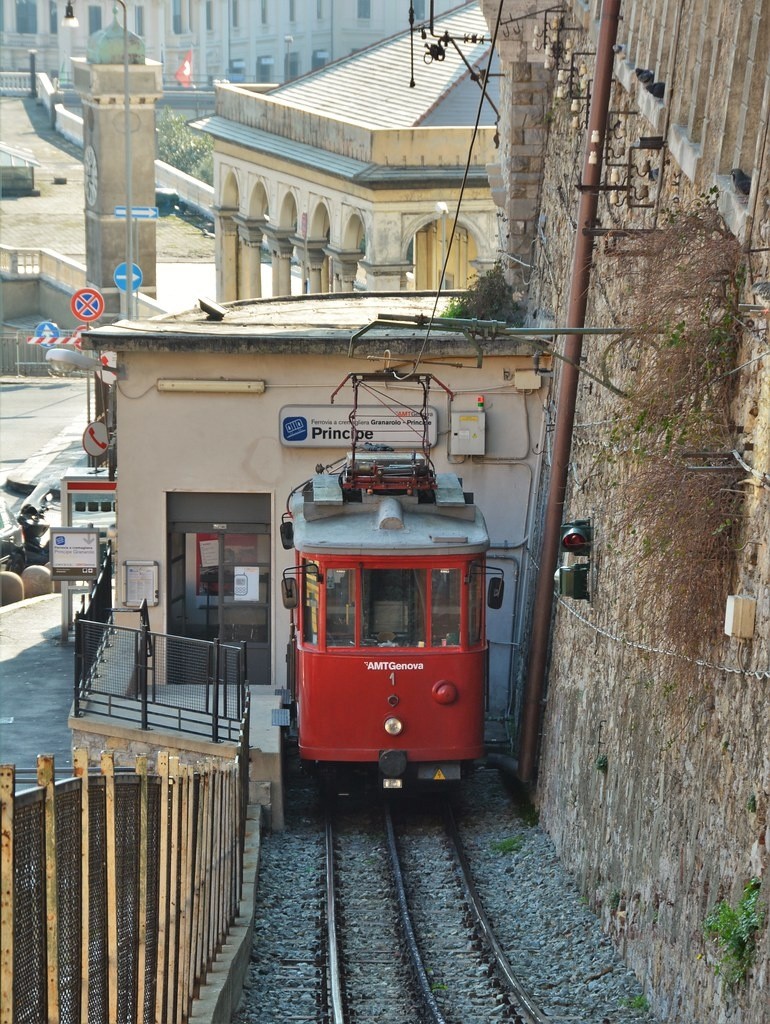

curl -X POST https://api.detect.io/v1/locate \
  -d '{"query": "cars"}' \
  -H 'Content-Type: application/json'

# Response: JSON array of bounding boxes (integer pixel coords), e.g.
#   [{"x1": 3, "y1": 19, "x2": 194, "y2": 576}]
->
[{"x1": 0, "y1": 495, "x2": 26, "y2": 561}]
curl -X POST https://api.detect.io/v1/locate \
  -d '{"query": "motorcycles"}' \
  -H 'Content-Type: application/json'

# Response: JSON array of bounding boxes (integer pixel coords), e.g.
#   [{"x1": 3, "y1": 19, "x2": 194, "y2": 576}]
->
[{"x1": 17, "y1": 493, "x2": 55, "y2": 562}]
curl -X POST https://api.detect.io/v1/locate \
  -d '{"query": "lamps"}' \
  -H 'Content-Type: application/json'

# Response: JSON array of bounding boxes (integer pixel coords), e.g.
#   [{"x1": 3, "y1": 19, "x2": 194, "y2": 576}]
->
[{"x1": 198, "y1": 299, "x2": 229, "y2": 320}]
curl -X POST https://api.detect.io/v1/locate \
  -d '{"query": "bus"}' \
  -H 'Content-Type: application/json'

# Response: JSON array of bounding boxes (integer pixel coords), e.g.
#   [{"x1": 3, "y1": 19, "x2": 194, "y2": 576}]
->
[{"x1": 268, "y1": 363, "x2": 492, "y2": 814}]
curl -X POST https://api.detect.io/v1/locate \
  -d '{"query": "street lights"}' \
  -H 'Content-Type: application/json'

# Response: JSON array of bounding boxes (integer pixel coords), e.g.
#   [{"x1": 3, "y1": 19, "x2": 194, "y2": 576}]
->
[{"x1": 284, "y1": 35, "x2": 293, "y2": 85}]
